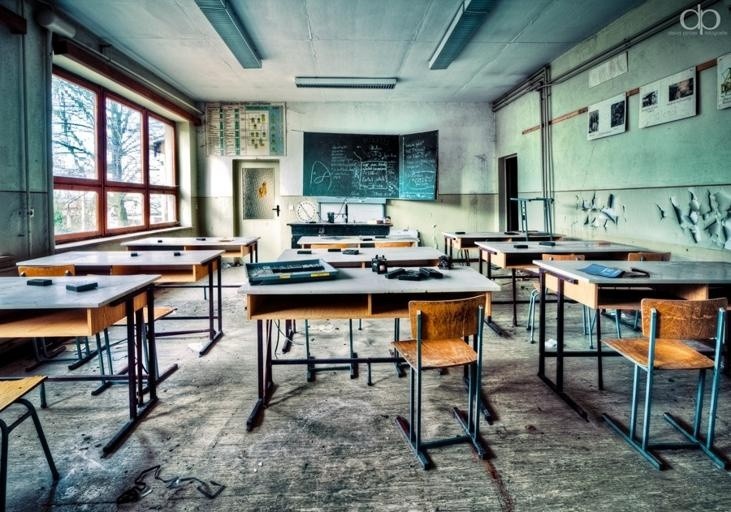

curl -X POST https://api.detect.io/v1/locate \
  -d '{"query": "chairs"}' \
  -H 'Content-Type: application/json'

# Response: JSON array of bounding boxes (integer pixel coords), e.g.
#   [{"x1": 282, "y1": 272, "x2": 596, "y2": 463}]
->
[
  {"x1": 0, "y1": 375, "x2": 62, "y2": 512},
  {"x1": 592, "y1": 252, "x2": 670, "y2": 339},
  {"x1": 18, "y1": 265, "x2": 106, "y2": 370},
  {"x1": 308, "y1": 243, "x2": 363, "y2": 331},
  {"x1": 510, "y1": 197, "x2": 554, "y2": 273},
  {"x1": 597, "y1": 297, "x2": 728, "y2": 473},
  {"x1": 374, "y1": 242, "x2": 416, "y2": 248},
  {"x1": 90, "y1": 306, "x2": 178, "y2": 397},
  {"x1": 527, "y1": 253, "x2": 594, "y2": 350},
  {"x1": 388, "y1": 293, "x2": 487, "y2": 470}
]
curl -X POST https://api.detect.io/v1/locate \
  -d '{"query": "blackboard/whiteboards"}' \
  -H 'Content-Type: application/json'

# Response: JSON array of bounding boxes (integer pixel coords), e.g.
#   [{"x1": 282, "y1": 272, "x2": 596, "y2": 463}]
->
[{"x1": 302, "y1": 130, "x2": 439, "y2": 202}]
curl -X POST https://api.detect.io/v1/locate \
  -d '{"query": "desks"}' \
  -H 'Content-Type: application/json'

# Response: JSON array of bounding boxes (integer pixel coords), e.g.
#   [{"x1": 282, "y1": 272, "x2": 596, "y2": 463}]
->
[
  {"x1": 120, "y1": 236, "x2": 260, "y2": 311},
  {"x1": 237, "y1": 235, "x2": 501, "y2": 439},
  {"x1": 16, "y1": 251, "x2": 227, "y2": 372},
  {"x1": 444, "y1": 231, "x2": 731, "y2": 426},
  {"x1": 1, "y1": 273, "x2": 162, "y2": 454}
]
